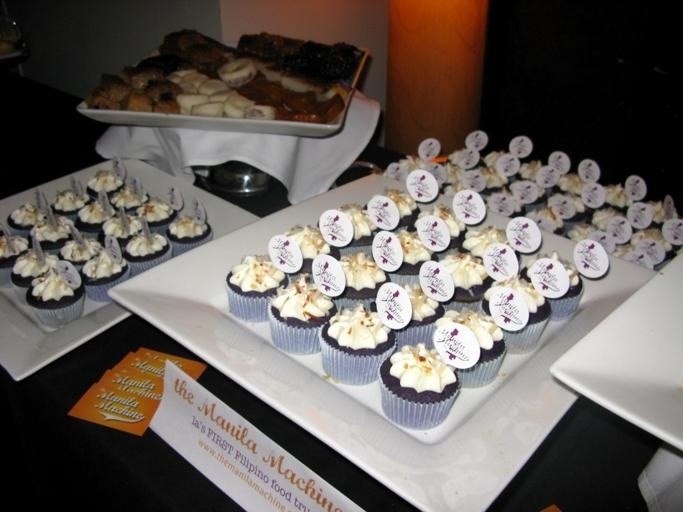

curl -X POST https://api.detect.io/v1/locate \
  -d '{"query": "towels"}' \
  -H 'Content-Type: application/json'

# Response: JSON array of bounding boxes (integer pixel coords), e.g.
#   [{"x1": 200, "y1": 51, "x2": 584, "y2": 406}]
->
[{"x1": 93, "y1": 84, "x2": 380, "y2": 204}]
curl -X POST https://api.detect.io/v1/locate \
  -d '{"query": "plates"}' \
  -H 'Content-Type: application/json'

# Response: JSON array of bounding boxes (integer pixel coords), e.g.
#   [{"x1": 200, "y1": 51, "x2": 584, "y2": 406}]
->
[
  {"x1": 76, "y1": 45, "x2": 372, "y2": 140},
  {"x1": 0, "y1": 156, "x2": 262, "y2": 386},
  {"x1": 105, "y1": 172, "x2": 660, "y2": 512},
  {"x1": 549, "y1": 253, "x2": 683, "y2": 449}
]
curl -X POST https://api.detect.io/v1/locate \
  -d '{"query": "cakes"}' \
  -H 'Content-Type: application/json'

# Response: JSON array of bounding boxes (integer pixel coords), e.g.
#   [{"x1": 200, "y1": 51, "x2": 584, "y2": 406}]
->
[
  {"x1": 0, "y1": 168, "x2": 212, "y2": 322},
  {"x1": 89, "y1": 27, "x2": 366, "y2": 125}
]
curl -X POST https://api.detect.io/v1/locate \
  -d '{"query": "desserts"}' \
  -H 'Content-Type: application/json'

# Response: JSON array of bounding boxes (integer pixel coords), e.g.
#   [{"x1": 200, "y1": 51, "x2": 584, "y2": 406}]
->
[
  {"x1": 382, "y1": 149, "x2": 680, "y2": 272},
  {"x1": 225, "y1": 187, "x2": 583, "y2": 429}
]
